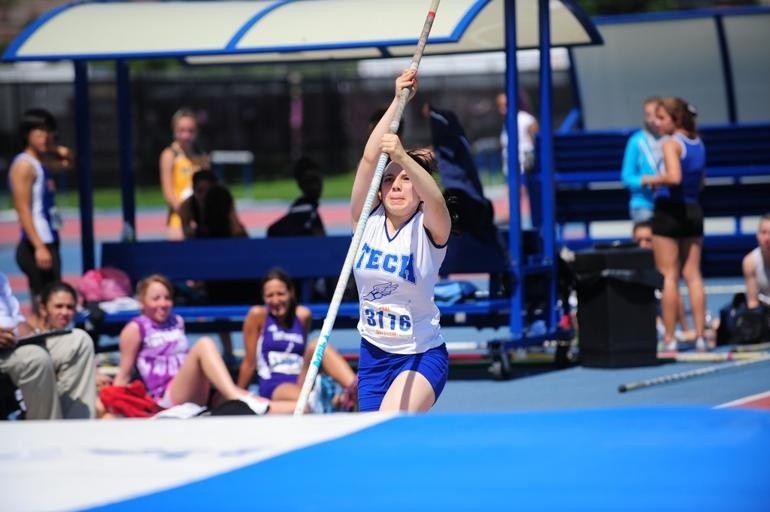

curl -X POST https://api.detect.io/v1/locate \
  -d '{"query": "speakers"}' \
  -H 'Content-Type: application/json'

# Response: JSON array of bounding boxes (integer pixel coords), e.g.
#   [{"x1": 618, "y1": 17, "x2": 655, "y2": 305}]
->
[{"x1": 573, "y1": 247, "x2": 658, "y2": 369}]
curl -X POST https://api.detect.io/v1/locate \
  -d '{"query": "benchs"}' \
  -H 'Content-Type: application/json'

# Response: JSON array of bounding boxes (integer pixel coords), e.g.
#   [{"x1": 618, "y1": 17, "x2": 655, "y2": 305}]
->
[
  {"x1": 527, "y1": 120, "x2": 769, "y2": 223},
  {"x1": 93, "y1": 228, "x2": 559, "y2": 373}
]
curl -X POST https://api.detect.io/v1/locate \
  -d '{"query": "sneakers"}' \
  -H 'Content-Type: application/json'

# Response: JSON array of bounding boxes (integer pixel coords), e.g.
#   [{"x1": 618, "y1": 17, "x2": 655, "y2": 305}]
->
[
  {"x1": 694, "y1": 335, "x2": 707, "y2": 352},
  {"x1": 307, "y1": 374, "x2": 331, "y2": 413},
  {"x1": 342, "y1": 373, "x2": 358, "y2": 410},
  {"x1": 238, "y1": 391, "x2": 270, "y2": 415},
  {"x1": 657, "y1": 338, "x2": 678, "y2": 352}
]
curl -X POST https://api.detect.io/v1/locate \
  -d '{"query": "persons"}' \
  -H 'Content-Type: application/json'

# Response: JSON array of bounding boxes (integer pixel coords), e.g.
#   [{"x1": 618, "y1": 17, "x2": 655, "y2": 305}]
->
[
  {"x1": 155, "y1": 101, "x2": 218, "y2": 238},
  {"x1": 174, "y1": 169, "x2": 245, "y2": 245},
  {"x1": 8, "y1": 110, "x2": 75, "y2": 292},
  {"x1": 228, "y1": 271, "x2": 358, "y2": 413},
  {"x1": 112, "y1": 272, "x2": 272, "y2": 414},
  {"x1": 490, "y1": 92, "x2": 536, "y2": 218},
  {"x1": 344, "y1": 61, "x2": 454, "y2": 413},
  {"x1": 618, "y1": 96, "x2": 718, "y2": 355},
  {"x1": 741, "y1": 216, "x2": 770, "y2": 333},
  {"x1": 1, "y1": 277, "x2": 106, "y2": 419}
]
